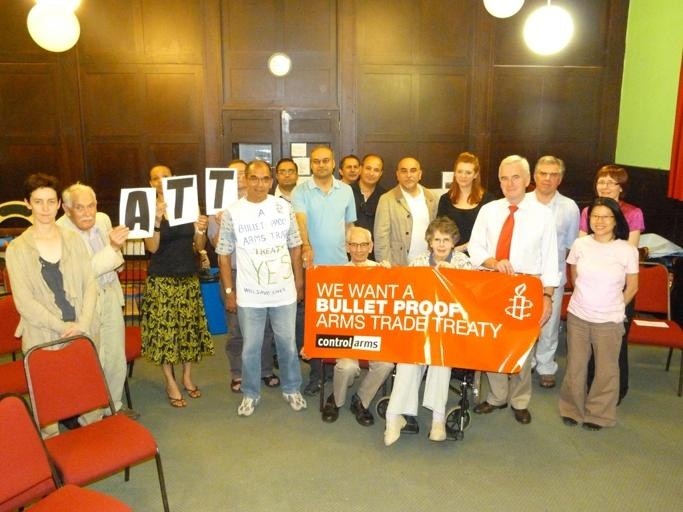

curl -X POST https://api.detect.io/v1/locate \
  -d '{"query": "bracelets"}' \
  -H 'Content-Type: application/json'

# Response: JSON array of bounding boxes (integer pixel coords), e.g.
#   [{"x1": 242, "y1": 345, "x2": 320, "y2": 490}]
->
[
  {"x1": 197, "y1": 228, "x2": 207, "y2": 235},
  {"x1": 154, "y1": 226, "x2": 161, "y2": 232}
]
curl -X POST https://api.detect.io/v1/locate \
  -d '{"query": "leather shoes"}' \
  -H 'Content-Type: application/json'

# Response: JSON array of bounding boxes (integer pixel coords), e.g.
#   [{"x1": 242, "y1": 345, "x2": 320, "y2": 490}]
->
[
  {"x1": 322, "y1": 393, "x2": 338, "y2": 422},
  {"x1": 473, "y1": 401, "x2": 508, "y2": 414},
  {"x1": 350, "y1": 394, "x2": 374, "y2": 426},
  {"x1": 511, "y1": 406, "x2": 531, "y2": 424}
]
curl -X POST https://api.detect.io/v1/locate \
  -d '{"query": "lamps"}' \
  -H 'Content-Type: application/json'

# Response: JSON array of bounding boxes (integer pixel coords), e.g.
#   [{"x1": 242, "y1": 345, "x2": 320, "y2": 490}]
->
[{"x1": 522, "y1": 0, "x2": 575, "y2": 56}]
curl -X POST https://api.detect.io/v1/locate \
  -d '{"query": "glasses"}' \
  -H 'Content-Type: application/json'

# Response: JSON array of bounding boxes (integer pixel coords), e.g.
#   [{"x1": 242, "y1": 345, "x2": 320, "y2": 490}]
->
[
  {"x1": 349, "y1": 242, "x2": 369, "y2": 248},
  {"x1": 592, "y1": 215, "x2": 615, "y2": 221},
  {"x1": 597, "y1": 180, "x2": 620, "y2": 187}
]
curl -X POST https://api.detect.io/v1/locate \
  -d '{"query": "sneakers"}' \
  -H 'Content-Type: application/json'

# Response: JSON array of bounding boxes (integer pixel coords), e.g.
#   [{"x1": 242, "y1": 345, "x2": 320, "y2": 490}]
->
[
  {"x1": 563, "y1": 417, "x2": 602, "y2": 431},
  {"x1": 119, "y1": 406, "x2": 140, "y2": 418},
  {"x1": 540, "y1": 374, "x2": 555, "y2": 388},
  {"x1": 383, "y1": 415, "x2": 407, "y2": 446},
  {"x1": 304, "y1": 376, "x2": 329, "y2": 396},
  {"x1": 282, "y1": 391, "x2": 307, "y2": 411},
  {"x1": 429, "y1": 421, "x2": 446, "y2": 442},
  {"x1": 238, "y1": 394, "x2": 261, "y2": 416}
]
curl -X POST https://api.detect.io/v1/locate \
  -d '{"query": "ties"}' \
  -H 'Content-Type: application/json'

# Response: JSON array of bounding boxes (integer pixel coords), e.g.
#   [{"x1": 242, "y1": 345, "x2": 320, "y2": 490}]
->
[{"x1": 495, "y1": 206, "x2": 519, "y2": 260}]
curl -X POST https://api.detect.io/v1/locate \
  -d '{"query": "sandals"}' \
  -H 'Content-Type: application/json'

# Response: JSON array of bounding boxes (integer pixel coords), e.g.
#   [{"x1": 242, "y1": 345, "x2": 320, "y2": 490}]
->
[
  {"x1": 263, "y1": 374, "x2": 280, "y2": 387},
  {"x1": 231, "y1": 379, "x2": 242, "y2": 393},
  {"x1": 166, "y1": 387, "x2": 187, "y2": 407},
  {"x1": 185, "y1": 385, "x2": 200, "y2": 398}
]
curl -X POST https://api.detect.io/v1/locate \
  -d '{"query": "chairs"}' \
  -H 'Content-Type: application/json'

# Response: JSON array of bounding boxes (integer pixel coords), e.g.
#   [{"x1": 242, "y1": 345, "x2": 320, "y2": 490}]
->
[
  {"x1": 24, "y1": 335, "x2": 170, "y2": 512},
  {"x1": 1, "y1": 293, "x2": 28, "y2": 396},
  {"x1": 0, "y1": 393, "x2": 133, "y2": 512},
  {"x1": 125, "y1": 326, "x2": 142, "y2": 408},
  {"x1": 561, "y1": 264, "x2": 573, "y2": 328},
  {"x1": 627, "y1": 262, "x2": 683, "y2": 396}
]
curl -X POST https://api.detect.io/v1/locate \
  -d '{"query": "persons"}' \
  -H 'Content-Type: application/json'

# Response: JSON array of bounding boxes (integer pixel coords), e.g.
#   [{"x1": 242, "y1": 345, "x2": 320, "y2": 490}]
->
[
  {"x1": 469, "y1": 155, "x2": 564, "y2": 425},
  {"x1": 143, "y1": 159, "x2": 208, "y2": 408},
  {"x1": 290, "y1": 144, "x2": 357, "y2": 396},
  {"x1": 508, "y1": 156, "x2": 580, "y2": 387},
  {"x1": 339, "y1": 155, "x2": 362, "y2": 183},
  {"x1": 436, "y1": 152, "x2": 498, "y2": 257},
  {"x1": 558, "y1": 196, "x2": 639, "y2": 431},
  {"x1": 193, "y1": 241, "x2": 210, "y2": 268},
  {"x1": 347, "y1": 153, "x2": 387, "y2": 262},
  {"x1": 6, "y1": 173, "x2": 102, "y2": 440},
  {"x1": 580, "y1": 165, "x2": 644, "y2": 406},
  {"x1": 383, "y1": 215, "x2": 475, "y2": 445},
  {"x1": 206, "y1": 159, "x2": 280, "y2": 393},
  {"x1": 322, "y1": 227, "x2": 395, "y2": 425},
  {"x1": 215, "y1": 159, "x2": 308, "y2": 416},
  {"x1": 54, "y1": 181, "x2": 142, "y2": 420},
  {"x1": 374, "y1": 155, "x2": 438, "y2": 266}
]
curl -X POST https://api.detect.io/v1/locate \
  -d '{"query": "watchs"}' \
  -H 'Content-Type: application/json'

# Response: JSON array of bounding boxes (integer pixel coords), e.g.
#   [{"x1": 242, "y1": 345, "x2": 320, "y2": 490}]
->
[
  {"x1": 543, "y1": 292, "x2": 554, "y2": 302},
  {"x1": 463, "y1": 245, "x2": 467, "y2": 251},
  {"x1": 225, "y1": 287, "x2": 235, "y2": 294}
]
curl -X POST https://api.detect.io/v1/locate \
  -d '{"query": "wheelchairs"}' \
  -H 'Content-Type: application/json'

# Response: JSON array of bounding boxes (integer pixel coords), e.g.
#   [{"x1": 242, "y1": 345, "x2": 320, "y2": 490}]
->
[{"x1": 374, "y1": 364, "x2": 484, "y2": 443}]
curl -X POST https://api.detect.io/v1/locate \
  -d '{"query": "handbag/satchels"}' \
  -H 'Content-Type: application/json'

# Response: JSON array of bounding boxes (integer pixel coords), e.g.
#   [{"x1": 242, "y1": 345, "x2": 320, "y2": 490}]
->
[{"x1": 194, "y1": 249, "x2": 210, "y2": 272}]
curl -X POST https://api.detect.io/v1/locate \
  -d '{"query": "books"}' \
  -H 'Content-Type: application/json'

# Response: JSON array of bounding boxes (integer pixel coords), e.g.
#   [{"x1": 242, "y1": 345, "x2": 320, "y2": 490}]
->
[{"x1": 117, "y1": 238, "x2": 150, "y2": 326}]
